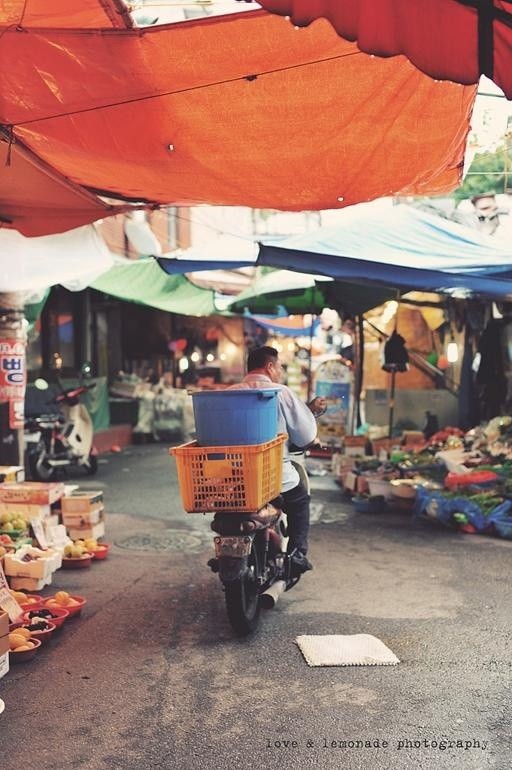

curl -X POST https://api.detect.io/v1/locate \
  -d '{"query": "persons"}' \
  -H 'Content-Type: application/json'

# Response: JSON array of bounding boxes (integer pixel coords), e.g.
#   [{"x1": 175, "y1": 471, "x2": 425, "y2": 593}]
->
[
  {"x1": 310, "y1": 397, "x2": 327, "y2": 412},
  {"x1": 224, "y1": 346, "x2": 317, "y2": 570}
]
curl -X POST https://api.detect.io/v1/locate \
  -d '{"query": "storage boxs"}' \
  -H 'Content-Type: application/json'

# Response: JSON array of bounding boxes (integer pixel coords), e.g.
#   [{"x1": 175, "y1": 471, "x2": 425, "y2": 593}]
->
[{"x1": 0, "y1": 480, "x2": 105, "y2": 541}]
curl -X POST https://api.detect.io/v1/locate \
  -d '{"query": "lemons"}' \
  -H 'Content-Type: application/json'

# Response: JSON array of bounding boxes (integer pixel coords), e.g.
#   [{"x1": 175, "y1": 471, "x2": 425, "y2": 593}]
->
[
  {"x1": 10, "y1": 589, "x2": 36, "y2": 605},
  {"x1": 64, "y1": 537, "x2": 104, "y2": 558}
]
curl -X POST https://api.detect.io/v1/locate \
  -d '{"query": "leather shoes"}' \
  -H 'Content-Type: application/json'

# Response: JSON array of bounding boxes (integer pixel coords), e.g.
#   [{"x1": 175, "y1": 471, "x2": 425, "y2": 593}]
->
[{"x1": 291, "y1": 551, "x2": 313, "y2": 570}]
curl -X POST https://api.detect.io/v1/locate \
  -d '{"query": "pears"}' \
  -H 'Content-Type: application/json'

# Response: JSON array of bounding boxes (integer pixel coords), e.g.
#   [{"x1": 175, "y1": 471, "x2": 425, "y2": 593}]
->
[{"x1": 0, "y1": 510, "x2": 27, "y2": 531}]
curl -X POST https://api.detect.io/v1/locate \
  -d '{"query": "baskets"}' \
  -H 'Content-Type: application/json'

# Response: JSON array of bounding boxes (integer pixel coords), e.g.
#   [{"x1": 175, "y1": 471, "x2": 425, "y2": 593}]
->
[{"x1": 168, "y1": 432, "x2": 288, "y2": 513}]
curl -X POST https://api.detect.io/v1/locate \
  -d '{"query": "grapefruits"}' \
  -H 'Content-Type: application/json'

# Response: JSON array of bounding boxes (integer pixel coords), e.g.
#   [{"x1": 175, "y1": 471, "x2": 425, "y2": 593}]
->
[{"x1": 9, "y1": 628, "x2": 35, "y2": 651}]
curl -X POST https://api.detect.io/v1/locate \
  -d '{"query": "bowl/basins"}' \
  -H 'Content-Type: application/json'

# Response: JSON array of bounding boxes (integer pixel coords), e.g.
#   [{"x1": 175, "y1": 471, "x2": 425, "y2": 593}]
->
[
  {"x1": 61, "y1": 552, "x2": 95, "y2": 569},
  {"x1": 352, "y1": 496, "x2": 394, "y2": 514},
  {"x1": 491, "y1": 515, "x2": 511, "y2": 537},
  {"x1": 389, "y1": 478, "x2": 425, "y2": 499},
  {"x1": 19, "y1": 593, "x2": 43, "y2": 610},
  {"x1": 367, "y1": 479, "x2": 395, "y2": 501},
  {"x1": 9, "y1": 620, "x2": 56, "y2": 643},
  {"x1": 7, "y1": 633, "x2": 42, "y2": 666},
  {"x1": 39, "y1": 594, "x2": 87, "y2": 619},
  {"x1": 19, "y1": 607, "x2": 70, "y2": 631},
  {"x1": 83, "y1": 543, "x2": 110, "y2": 561}
]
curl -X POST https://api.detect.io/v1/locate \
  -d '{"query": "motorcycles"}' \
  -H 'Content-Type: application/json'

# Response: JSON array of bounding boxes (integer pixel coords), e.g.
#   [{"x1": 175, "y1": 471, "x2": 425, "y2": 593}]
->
[
  {"x1": 207, "y1": 395, "x2": 329, "y2": 634},
  {"x1": 24, "y1": 360, "x2": 98, "y2": 482}
]
